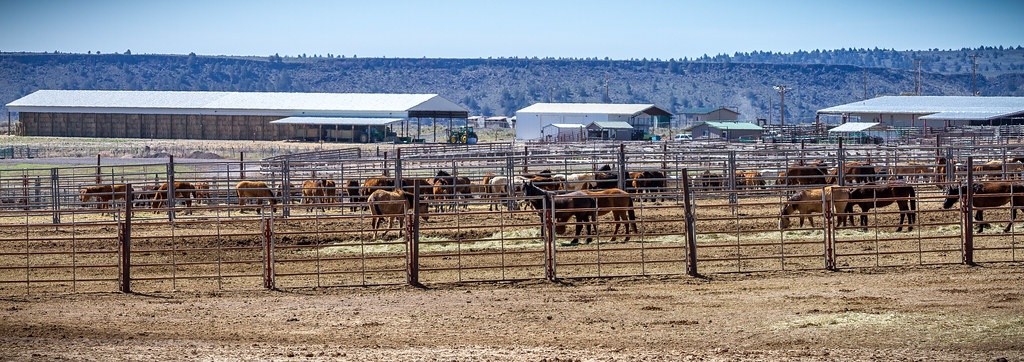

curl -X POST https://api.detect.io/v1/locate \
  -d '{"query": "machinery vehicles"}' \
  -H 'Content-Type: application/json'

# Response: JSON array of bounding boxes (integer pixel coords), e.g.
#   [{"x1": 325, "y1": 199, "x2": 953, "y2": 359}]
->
[
  {"x1": 446, "y1": 126, "x2": 478, "y2": 144},
  {"x1": 359, "y1": 127, "x2": 384, "y2": 143}
]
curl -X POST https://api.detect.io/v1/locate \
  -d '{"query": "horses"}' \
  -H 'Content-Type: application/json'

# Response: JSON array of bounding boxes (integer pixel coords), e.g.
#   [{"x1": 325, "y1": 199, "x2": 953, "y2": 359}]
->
[{"x1": 79, "y1": 160, "x2": 1023, "y2": 245}]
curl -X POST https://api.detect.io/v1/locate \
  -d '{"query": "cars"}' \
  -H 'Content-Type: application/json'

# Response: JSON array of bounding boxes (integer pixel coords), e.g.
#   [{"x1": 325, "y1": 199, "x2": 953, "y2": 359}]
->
[{"x1": 674, "y1": 134, "x2": 692, "y2": 140}]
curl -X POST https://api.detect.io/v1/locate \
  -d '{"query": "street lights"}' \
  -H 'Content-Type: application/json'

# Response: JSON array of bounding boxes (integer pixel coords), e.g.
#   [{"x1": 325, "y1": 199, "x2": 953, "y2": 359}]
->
[{"x1": 773, "y1": 84, "x2": 792, "y2": 143}]
